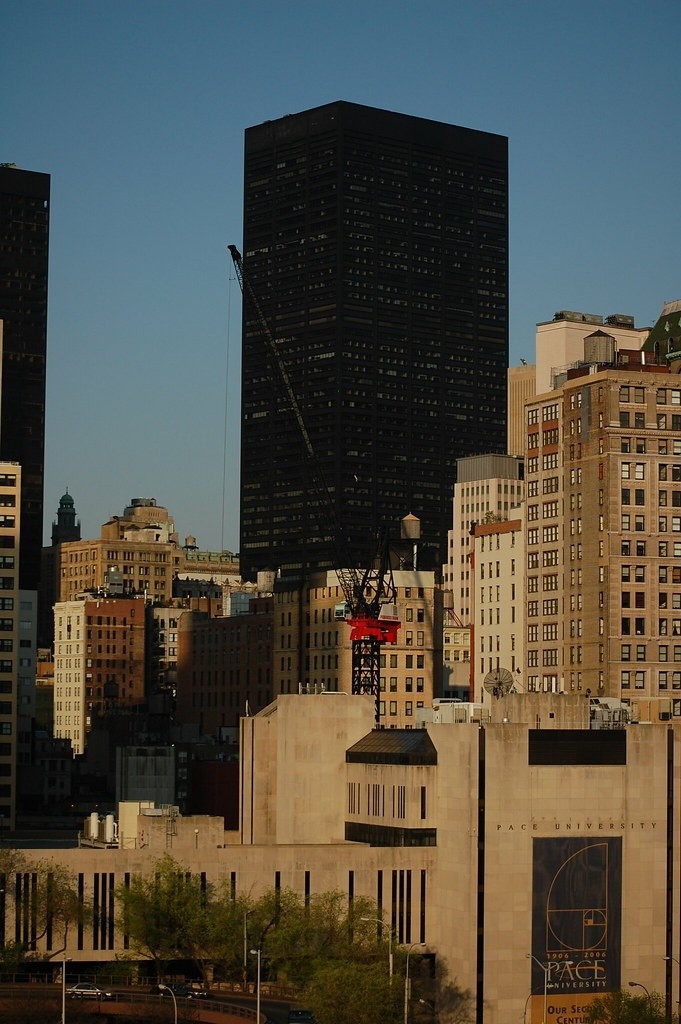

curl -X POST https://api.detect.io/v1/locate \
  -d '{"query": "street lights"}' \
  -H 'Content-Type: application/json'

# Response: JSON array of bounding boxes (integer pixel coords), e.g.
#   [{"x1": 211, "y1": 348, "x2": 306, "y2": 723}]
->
[
  {"x1": 230, "y1": 898, "x2": 247, "y2": 991},
  {"x1": 62, "y1": 952, "x2": 72, "y2": 1024},
  {"x1": 158, "y1": 984, "x2": 178, "y2": 1024},
  {"x1": 361, "y1": 917, "x2": 394, "y2": 993},
  {"x1": 403, "y1": 943, "x2": 427, "y2": 1022},
  {"x1": 250, "y1": 949, "x2": 260, "y2": 1024}
]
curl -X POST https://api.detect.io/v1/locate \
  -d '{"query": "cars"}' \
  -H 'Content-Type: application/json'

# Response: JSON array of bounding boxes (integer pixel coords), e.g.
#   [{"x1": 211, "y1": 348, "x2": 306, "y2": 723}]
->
[
  {"x1": 65, "y1": 982, "x2": 113, "y2": 1000},
  {"x1": 288, "y1": 1007, "x2": 316, "y2": 1024},
  {"x1": 149, "y1": 983, "x2": 209, "y2": 1000},
  {"x1": 62, "y1": 795, "x2": 99, "y2": 815}
]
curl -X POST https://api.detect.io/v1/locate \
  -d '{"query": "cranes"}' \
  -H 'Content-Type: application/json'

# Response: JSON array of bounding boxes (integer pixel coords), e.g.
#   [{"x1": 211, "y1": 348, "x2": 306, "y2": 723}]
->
[{"x1": 226, "y1": 241, "x2": 411, "y2": 728}]
